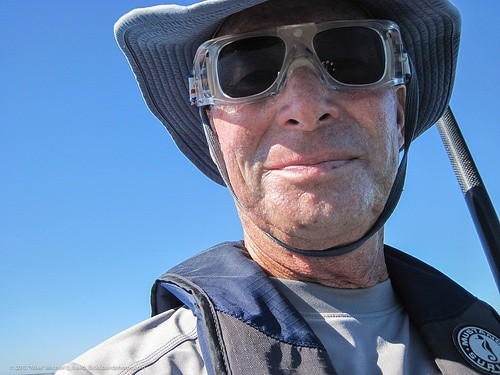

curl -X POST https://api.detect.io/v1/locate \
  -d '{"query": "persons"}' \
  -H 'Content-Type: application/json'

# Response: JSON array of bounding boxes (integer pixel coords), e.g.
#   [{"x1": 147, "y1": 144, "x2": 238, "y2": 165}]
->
[{"x1": 47, "y1": 1, "x2": 500, "y2": 374}]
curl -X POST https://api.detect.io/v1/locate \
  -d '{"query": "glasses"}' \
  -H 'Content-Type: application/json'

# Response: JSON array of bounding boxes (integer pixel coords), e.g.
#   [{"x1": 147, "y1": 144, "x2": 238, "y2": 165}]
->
[{"x1": 186, "y1": 17, "x2": 409, "y2": 105}]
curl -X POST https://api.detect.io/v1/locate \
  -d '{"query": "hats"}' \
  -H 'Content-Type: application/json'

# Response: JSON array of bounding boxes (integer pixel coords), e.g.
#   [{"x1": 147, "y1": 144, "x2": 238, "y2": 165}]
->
[{"x1": 114, "y1": 0, "x2": 463, "y2": 186}]
